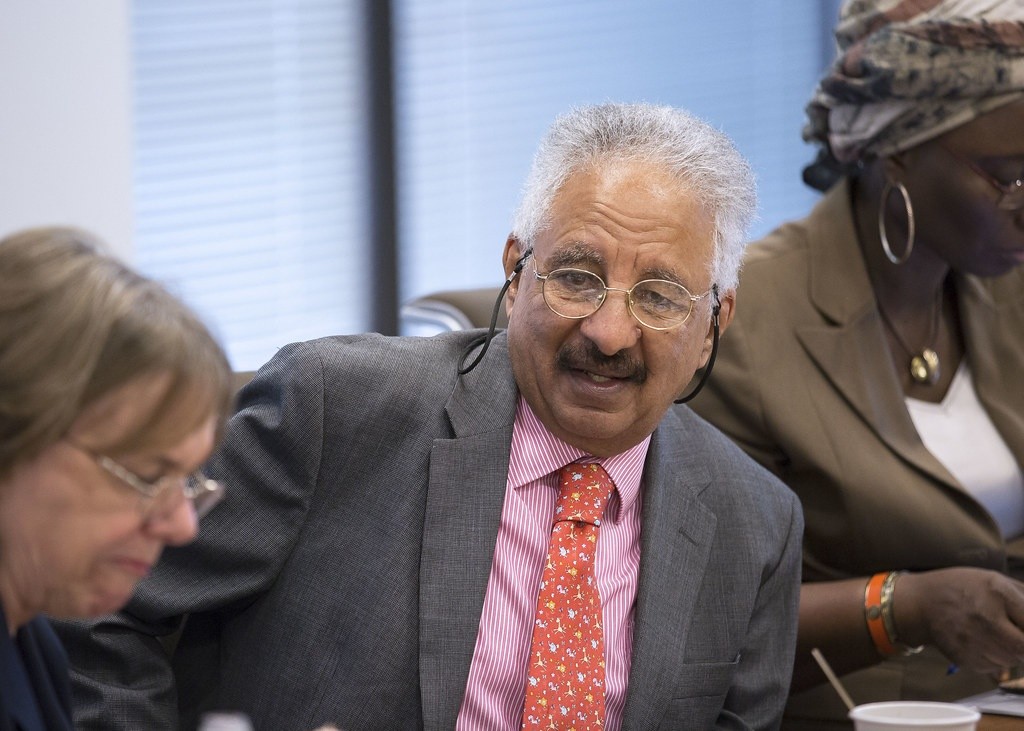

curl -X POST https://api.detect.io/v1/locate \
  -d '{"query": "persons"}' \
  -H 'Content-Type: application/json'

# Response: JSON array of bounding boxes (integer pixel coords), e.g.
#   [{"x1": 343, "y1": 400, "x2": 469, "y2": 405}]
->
[
  {"x1": 673, "y1": 0, "x2": 1024, "y2": 731},
  {"x1": 52, "y1": 102, "x2": 803, "y2": 731},
  {"x1": 0, "y1": 226, "x2": 235, "y2": 731}
]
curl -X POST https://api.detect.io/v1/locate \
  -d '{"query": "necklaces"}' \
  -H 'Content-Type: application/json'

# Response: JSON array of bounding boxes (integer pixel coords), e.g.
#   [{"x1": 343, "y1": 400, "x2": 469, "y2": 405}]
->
[{"x1": 869, "y1": 286, "x2": 940, "y2": 386}]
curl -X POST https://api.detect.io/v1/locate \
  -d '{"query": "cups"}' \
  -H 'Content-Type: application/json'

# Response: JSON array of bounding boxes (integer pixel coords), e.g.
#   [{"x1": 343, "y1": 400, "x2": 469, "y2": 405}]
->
[{"x1": 848, "y1": 700, "x2": 982, "y2": 731}]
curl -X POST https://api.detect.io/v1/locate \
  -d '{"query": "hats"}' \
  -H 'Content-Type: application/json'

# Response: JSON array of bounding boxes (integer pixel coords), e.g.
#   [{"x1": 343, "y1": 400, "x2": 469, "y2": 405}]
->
[{"x1": 798, "y1": 0, "x2": 1023, "y2": 192}]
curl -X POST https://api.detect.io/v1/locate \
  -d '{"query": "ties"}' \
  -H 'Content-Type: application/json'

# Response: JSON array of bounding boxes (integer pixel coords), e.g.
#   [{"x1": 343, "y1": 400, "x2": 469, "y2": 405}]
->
[{"x1": 523, "y1": 461, "x2": 615, "y2": 731}]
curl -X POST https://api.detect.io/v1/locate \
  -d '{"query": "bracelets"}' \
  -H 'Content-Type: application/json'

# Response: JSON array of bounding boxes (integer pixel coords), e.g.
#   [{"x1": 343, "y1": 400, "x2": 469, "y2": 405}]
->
[{"x1": 864, "y1": 571, "x2": 924, "y2": 662}]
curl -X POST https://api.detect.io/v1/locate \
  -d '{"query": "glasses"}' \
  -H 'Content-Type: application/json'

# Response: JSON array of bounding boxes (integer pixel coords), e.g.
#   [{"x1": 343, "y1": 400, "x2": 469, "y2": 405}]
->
[
  {"x1": 528, "y1": 245, "x2": 722, "y2": 330},
  {"x1": 58, "y1": 428, "x2": 224, "y2": 519},
  {"x1": 930, "y1": 135, "x2": 1023, "y2": 212}
]
